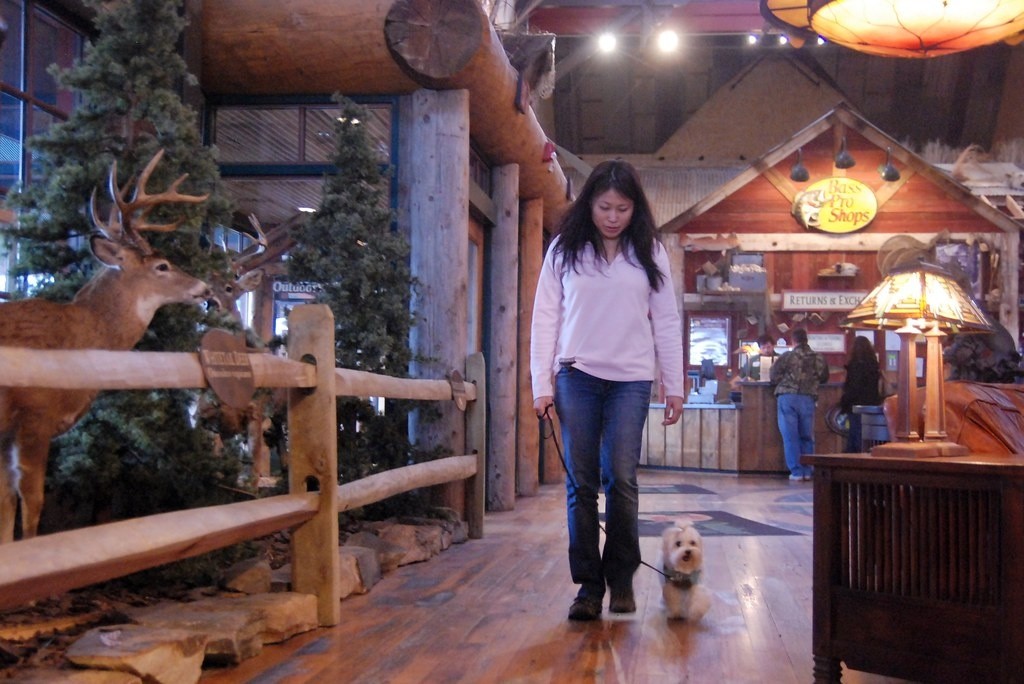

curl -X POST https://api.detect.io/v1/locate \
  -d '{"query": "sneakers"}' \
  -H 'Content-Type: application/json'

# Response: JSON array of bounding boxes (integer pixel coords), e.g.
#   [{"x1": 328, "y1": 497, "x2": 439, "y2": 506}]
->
[
  {"x1": 569, "y1": 581, "x2": 606, "y2": 617},
  {"x1": 610, "y1": 583, "x2": 635, "y2": 613}
]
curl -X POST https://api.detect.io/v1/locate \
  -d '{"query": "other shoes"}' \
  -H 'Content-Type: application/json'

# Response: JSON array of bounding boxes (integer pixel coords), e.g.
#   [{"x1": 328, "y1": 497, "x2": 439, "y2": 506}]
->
[
  {"x1": 789, "y1": 474, "x2": 803, "y2": 481},
  {"x1": 803, "y1": 476, "x2": 810, "y2": 480}
]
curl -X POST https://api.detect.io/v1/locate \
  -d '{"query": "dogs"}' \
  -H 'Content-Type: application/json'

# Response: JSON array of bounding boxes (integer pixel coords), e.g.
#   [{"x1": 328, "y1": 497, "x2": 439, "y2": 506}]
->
[{"x1": 656, "y1": 528, "x2": 713, "y2": 626}]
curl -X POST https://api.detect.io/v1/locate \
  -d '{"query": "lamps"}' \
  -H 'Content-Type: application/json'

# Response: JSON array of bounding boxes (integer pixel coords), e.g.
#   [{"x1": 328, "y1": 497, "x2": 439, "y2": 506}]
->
[
  {"x1": 836, "y1": 256, "x2": 995, "y2": 454},
  {"x1": 791, "y1": 148, "x2": 810, "y2": 182},
  {"x1": 879, "y1": 146, "x2": 901, "y2": 181},
  {"x1": 835, "y1": 137, "x2": 856, "y2": 169}
]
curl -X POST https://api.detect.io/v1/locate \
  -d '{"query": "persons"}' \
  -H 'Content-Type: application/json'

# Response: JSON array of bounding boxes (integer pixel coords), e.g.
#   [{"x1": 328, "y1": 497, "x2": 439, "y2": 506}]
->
[
  {"x1": 531, "y1": 160, "x2": 687, "y2": 621},
  {"x1": 769, "y1": 329, "x2": 830, "y2": 482},
  {"x1": 943, "y1": 313, "x2": 1024, "y2": 455},
  {"x1": 839, "y1": 335, "x2": 887, "y2": 453},
  {"x1": 729, "y1": 334, "x2": 781, "y2": 390}
]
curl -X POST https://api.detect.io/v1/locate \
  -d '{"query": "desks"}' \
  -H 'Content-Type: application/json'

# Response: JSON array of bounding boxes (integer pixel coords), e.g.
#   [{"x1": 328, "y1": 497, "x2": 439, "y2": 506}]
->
[{"x1": 798, "y1": 452, "x2": 1024, "y2": 684}]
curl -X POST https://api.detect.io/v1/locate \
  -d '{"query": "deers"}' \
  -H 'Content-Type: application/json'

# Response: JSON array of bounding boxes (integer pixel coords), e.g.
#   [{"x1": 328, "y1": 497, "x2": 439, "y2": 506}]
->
[
  {"x1": 186, "y1": 211, "x2": 263, "y2": 498},
  {"x1": 0, "y1": 150, "x2": 216, "y2": 611}
]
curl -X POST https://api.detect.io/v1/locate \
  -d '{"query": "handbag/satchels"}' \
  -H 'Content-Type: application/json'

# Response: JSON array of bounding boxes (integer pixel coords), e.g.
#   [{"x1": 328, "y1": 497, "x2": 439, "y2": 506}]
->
[{"x1": 878, "y1": 370, "x2": 895, "y2": 399}]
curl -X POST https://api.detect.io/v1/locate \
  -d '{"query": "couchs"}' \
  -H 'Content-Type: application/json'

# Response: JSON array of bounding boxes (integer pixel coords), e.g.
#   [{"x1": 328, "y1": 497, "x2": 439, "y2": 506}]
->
[{"x1": 883, "y1": 378, "x2": 1024, "y2": 456}]
